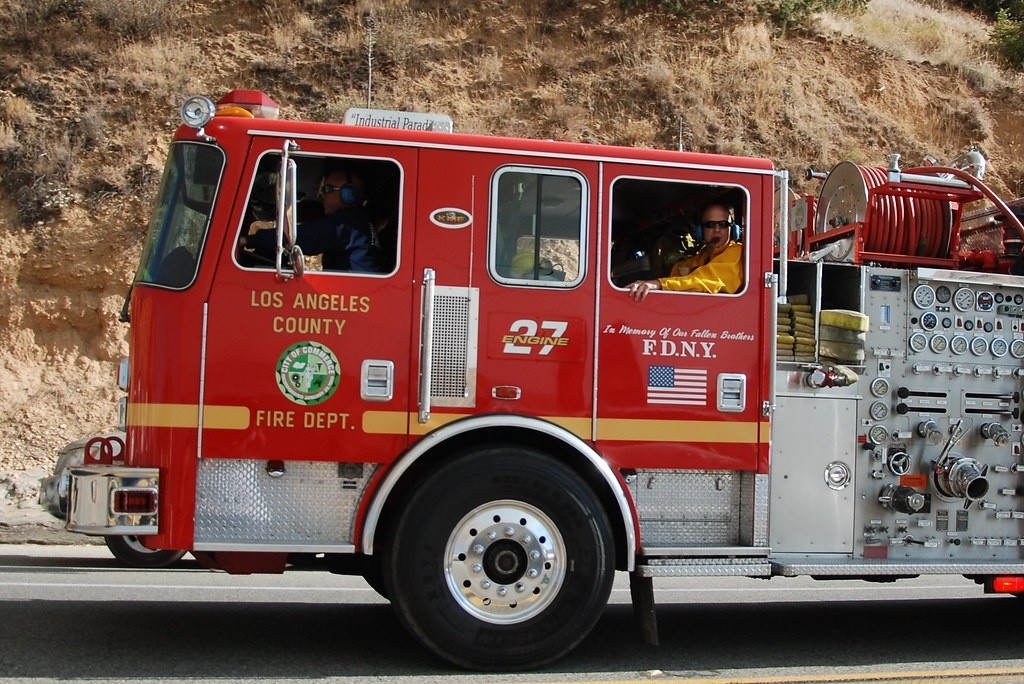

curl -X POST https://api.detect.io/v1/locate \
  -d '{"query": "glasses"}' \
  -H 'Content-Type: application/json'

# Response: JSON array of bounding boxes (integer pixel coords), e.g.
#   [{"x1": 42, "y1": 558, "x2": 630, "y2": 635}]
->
[
  {"x1": 321, "y1": 184, "x2": 342, "y2": 194},
  {"x1": 702, "y1": 220, "x2": 733, "y2": 228}
]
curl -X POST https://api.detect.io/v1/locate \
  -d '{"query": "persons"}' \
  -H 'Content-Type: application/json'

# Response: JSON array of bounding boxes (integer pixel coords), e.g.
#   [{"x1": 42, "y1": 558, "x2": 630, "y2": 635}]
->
[
  {"x1": 182, "y1": 162, "x2": 384, "y2": 274},
  {"x1": 624, "y1": 204, "x2": 743, "y2": 302}
]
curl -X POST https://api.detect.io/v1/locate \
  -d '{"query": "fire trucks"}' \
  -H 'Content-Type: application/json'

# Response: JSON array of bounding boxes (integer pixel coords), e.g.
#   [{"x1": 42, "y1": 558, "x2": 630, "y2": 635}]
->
[{"x1": 35, "y1": 90, "x2": 1024, "y2": 674}]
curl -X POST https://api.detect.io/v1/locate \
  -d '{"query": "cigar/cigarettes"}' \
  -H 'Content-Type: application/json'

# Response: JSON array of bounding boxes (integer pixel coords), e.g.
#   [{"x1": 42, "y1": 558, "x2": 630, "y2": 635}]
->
[{"x1": 707, "y1": 240, "x2": 718, "y2": 246}]
